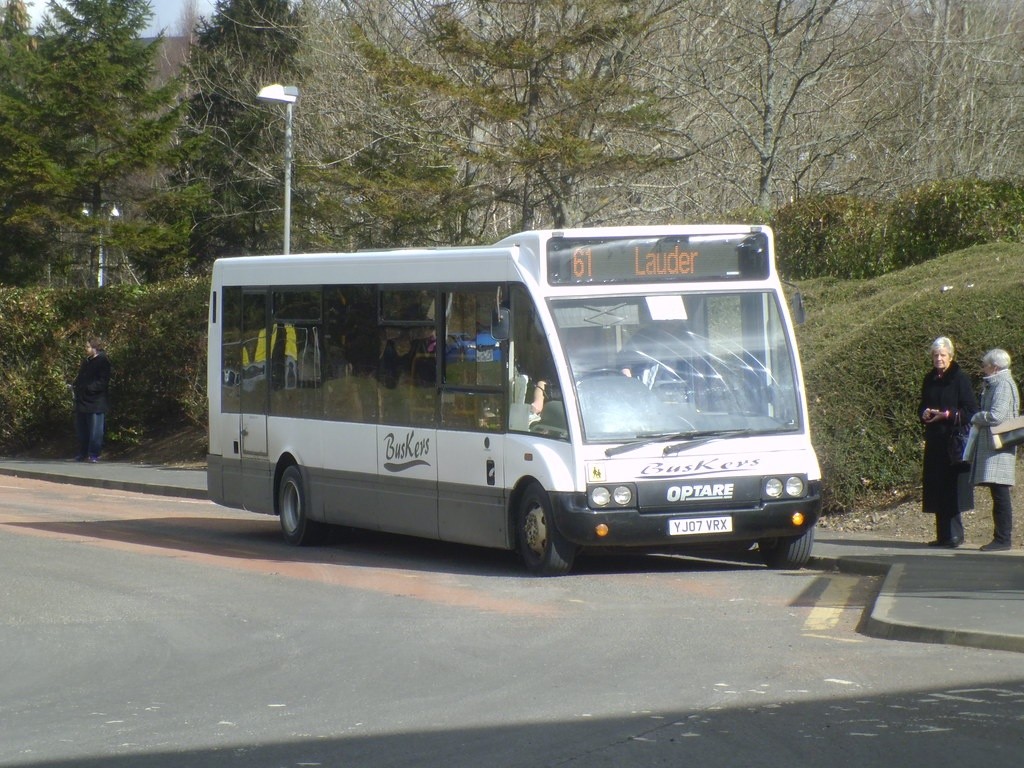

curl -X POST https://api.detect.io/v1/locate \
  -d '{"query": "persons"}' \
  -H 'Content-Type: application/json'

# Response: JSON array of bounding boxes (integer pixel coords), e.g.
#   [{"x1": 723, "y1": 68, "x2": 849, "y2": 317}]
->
[
  {"x1": 615, "y1": 319, "x2": 688, "y2": 405},
  {"x1": 529, "y1": 336, "x2": 592, "y2": 415},
  {"x1": 916, "y1": 337, "x2": 978, "y2": 547},
  {"x1": 64, "y1": 337, "x2": 111, "y2": 463},
  {"x1": 972, "y1": 349, "x2": 1020, "y2": 552}
]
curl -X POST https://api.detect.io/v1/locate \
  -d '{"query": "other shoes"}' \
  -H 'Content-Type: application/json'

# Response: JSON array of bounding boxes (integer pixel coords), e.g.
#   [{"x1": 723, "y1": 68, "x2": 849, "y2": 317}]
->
[
  {"x1": 980, "y1": 541, "x2": 1010, "y2": 551},
  {"x1": 948, "y1": 536, "x2": 964, "y2": 548},
  {"x1": 76, "y1": 456, "x2": 86, "y2": 463},
  {"x1": 928, "y1": 540, "x2": 949, "y2": 546},
  {"x1": 90, "y1": 457, "x2": 97, "y2": 463}
]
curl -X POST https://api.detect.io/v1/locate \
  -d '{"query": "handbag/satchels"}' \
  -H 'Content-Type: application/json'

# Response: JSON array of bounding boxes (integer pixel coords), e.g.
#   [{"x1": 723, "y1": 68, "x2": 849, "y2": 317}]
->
[
  {"x1": 949, "y1": 412, "x2": 971, "y2": 466},
  {"x1": 989, "y1": 380, "x2": 1024, "y2": 450},
  {"x1": 962, "y1": 423, "x2": 977, "y2": 464}
]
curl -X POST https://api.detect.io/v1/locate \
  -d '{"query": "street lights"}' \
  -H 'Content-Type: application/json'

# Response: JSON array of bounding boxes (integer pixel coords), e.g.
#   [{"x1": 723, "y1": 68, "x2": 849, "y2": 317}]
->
[
  {"x1": 80, "y1": 201, "x2": 121, "y2": 288},
  {"x1": 254, "y1": 81, "x2": 298, "y2": 254}
]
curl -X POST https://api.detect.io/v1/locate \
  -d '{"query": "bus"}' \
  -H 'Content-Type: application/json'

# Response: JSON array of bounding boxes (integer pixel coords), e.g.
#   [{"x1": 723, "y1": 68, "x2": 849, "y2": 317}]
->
[{"x1": 205, "y1": 222, "x2": 826, "y2": 576}]
